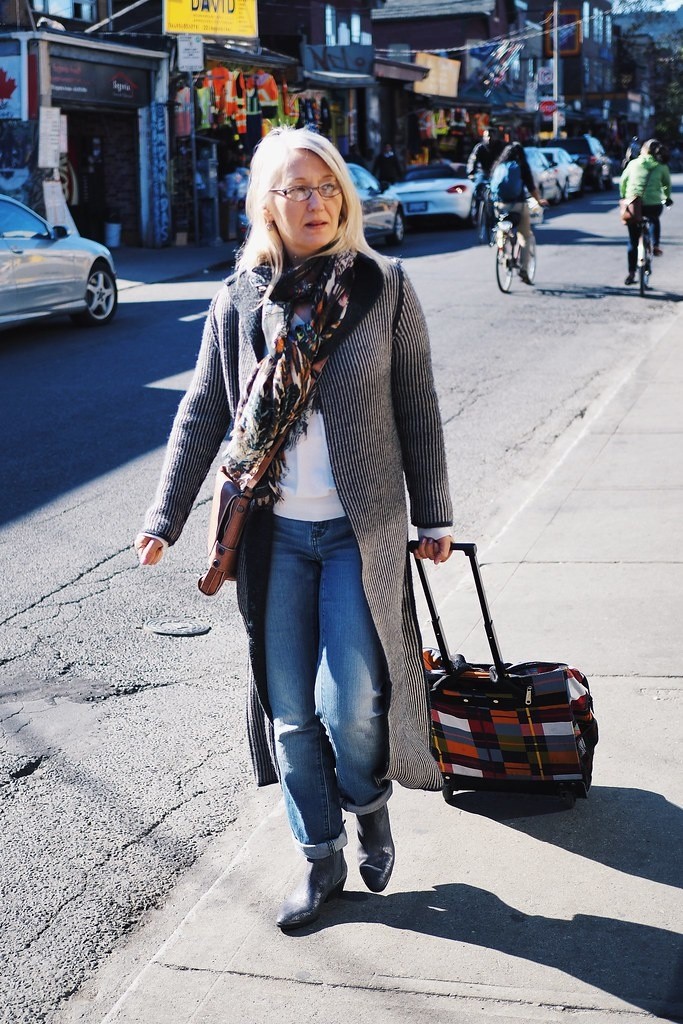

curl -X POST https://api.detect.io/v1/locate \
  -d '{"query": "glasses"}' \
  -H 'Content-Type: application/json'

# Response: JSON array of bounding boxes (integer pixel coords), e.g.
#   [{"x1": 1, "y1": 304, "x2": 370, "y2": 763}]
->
[{"x1": 269, "y1": 183, "x2": 342, "y2": 202}]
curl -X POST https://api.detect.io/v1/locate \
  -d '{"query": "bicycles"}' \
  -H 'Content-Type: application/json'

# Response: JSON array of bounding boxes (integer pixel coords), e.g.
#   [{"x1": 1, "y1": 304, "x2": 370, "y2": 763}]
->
[
  {"x1": 638, "y1": 200, "x2": 671, "y2": 297},
  {"x1": 493, "y1": 202, "x2": 548, "y2": 293}
]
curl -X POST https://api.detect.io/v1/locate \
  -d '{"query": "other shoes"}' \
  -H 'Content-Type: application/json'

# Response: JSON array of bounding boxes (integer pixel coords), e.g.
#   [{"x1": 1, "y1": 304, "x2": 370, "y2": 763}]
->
[
  {"x1": 518, "y1": 270, "x2": 531, "y2": 285},
  {"x1": 625, "y1": 273, "x2": 634, "y2": 285},
  {"x1": 653, "y1": 249, "x2": 663, "y2": 257}
]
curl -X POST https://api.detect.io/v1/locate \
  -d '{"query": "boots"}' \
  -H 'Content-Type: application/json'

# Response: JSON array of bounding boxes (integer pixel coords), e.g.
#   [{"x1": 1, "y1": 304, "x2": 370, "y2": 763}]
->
[
  {"x1": 276, "y1": 848, "x2": 347, "y2": 930},
  {"x1": 356, "y1": 804, "x2": 395, "y2": 892}
]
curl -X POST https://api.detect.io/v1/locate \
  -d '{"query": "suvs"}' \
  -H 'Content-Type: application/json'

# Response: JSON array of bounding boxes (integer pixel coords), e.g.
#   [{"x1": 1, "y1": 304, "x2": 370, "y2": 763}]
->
[{"x1": 549, "y1": 135, "x2": 612, "y2": 192}]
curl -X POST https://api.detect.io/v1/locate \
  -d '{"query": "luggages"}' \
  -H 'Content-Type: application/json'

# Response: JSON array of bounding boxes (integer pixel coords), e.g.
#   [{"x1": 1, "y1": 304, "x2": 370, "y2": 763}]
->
[{"x1": 408, "y1": 540, "x2": 599, "y2": 807}]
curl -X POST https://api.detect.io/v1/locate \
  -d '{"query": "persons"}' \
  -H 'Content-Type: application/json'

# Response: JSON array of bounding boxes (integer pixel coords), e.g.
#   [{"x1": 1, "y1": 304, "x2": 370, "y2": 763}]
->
[
  {"x1": 373, "y1": 143, "x2": 399, "y2": 187},
  {"x1": 626, "y1": 137, "x2": 641, "y2": 162},
  {"x1": 467, "y1": 129, "x2": 548, "y2": 284},
  {"x1": 620, "y1": 140, "x2": 673, "y2": 284},
  {"x1": 136, "y1": 129, "x2": 452, "y2": 927}
]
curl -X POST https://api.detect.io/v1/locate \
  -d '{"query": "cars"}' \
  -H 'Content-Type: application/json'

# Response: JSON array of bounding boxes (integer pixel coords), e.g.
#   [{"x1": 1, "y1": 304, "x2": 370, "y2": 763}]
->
[
  {"x1": 237, "y1": 162, "x2": 405, "y2": 247},
  {"x1": 381, "y1": 162, "x2": 477, "y2": 228},
  {"x1": 0, "y1": 193, "x2": 118, "y2": 334},
  {"x1": 525, "y1": 146, "x2": 584, "y2": 206}
]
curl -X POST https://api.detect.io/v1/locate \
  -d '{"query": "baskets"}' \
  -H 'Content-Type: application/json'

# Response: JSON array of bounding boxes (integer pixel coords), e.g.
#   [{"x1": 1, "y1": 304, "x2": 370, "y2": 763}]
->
[{"x1": 530, "y1": 205, "x2": 544, "y2": 224}]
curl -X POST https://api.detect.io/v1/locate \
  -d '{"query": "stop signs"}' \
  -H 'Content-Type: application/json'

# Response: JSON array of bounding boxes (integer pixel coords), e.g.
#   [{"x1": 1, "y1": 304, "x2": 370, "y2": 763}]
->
[{"x1": 539, "y1": 101, "x2": 556, "y2": 114}]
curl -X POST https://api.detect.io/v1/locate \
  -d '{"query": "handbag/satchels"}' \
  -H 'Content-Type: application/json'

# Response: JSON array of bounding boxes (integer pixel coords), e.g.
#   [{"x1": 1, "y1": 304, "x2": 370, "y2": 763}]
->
[
  {"x1": 619, "y1": 197, "x2": 642, "y2": 224},
  {"x1": 197, "y1": 466, "x2": 251, "y2": 596}
]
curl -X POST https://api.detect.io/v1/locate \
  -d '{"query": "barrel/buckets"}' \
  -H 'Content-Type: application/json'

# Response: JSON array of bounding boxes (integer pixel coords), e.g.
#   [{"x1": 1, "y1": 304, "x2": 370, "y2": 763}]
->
[{"x1": 105, "y1": 223, "x2": 121, "y2": 248}]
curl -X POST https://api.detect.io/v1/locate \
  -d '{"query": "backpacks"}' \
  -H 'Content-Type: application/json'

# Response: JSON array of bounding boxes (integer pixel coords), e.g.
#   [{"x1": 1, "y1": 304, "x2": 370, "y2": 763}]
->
[{"x1": 491, "y1": 160, "x2": 522, "y2": 201}]
